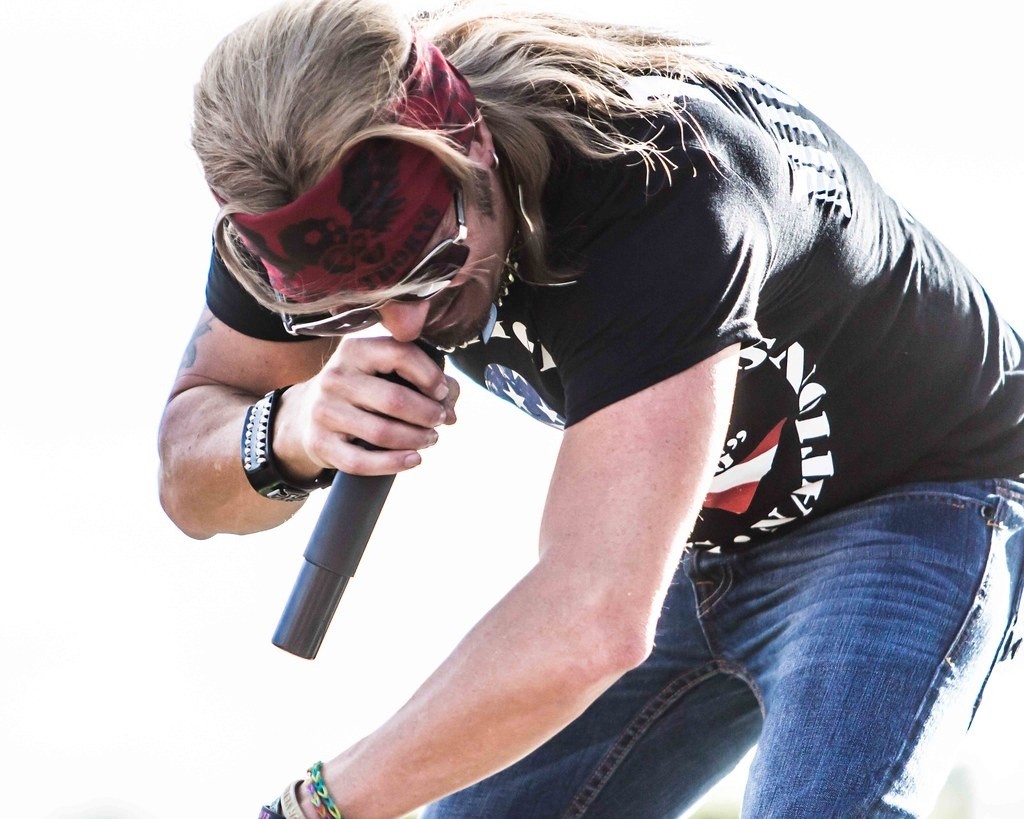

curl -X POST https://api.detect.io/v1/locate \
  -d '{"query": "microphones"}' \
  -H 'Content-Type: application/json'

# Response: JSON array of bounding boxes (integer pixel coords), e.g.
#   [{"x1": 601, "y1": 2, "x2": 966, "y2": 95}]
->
[{"x1": 272, "y1": 335, "x2": 445, "y2": 659}]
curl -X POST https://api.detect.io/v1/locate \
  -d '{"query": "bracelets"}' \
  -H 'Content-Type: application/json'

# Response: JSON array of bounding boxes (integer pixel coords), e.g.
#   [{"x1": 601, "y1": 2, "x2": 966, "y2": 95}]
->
[
  {"x1": 240, "y1": 385, "x2": 334, "y2": 505},
  {"x1": 257, "y1": 759, "x2": 342, "y2": 819}
]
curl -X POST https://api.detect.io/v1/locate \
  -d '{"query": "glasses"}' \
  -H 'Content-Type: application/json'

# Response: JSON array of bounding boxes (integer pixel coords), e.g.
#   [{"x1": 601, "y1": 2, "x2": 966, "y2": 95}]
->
[{"x1": 273, "y1": 180, "x2": 470, "y2": 337}]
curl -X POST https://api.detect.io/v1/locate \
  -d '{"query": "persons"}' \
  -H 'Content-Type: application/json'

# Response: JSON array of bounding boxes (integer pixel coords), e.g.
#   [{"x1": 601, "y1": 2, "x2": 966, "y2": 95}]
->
[{"x1": 157, "y1": 2, "x2": 1024, "y2": 818}]
